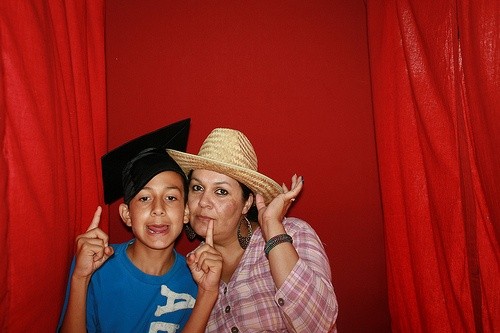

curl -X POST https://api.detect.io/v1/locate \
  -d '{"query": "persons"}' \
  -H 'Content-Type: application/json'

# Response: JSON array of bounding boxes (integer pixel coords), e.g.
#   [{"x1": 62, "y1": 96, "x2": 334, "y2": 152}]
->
[
  {"x1": 54, "y1": 118, "x2": 223, "y2": 333},
  {"x1": 165, "y1": 128, "x2": 339, "y2": 333}
]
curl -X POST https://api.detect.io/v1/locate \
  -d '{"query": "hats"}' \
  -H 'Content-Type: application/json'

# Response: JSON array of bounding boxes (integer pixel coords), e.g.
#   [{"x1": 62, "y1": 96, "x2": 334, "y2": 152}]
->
[
  {"x1": 165, "y1": 127, "x2": 283, "y2": 206},
  {"x1": 101, "y1": 118, "x2": 190, "y2": 206}
]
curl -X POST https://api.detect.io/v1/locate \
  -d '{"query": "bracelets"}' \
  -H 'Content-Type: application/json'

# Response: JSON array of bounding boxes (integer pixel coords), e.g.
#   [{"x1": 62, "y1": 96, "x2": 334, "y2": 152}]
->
[{"x1": 264, "y1": 235, "x2": 292, "y2": 254}]
